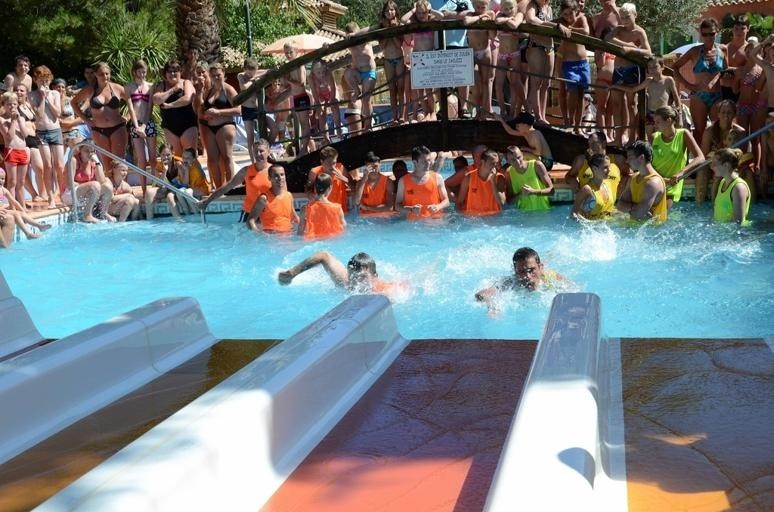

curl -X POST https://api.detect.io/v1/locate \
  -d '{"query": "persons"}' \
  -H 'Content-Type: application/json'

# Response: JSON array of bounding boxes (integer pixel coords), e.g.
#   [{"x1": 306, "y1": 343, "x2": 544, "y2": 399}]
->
[
  {"x1": 1, "y1": 1, "x2": 774, "y2": 247},
  {"x1": 474, "y1": 248, "x2": 580, "y2": 320},
  {"x1": 277, "y1": 251, "x2": 410, "y2": 304}
]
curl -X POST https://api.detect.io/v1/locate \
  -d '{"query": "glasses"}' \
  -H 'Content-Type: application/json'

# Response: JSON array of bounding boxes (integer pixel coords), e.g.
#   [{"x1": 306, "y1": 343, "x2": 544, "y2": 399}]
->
[{"x1": 702, "y1": 33, "x2": 715, "y2": 36}]
